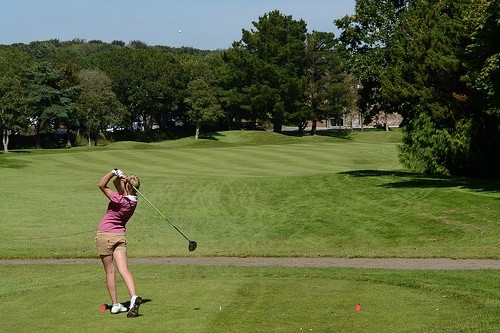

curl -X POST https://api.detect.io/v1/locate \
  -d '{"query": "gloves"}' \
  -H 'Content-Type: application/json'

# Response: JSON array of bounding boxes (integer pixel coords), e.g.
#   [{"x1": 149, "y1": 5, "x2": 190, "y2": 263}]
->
[
  {"x1": 112, "y1": 169, "x2": 122, "y2": 175},
  {"x1": 117, "y1": 172, "x2": 126, "y2": 178}
]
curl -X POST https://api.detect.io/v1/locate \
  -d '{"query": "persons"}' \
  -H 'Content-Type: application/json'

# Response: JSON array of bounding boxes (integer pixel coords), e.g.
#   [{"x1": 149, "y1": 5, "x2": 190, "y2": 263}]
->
[{"x1": 95, "y1": 168, "x2": 142, "y2": 317}]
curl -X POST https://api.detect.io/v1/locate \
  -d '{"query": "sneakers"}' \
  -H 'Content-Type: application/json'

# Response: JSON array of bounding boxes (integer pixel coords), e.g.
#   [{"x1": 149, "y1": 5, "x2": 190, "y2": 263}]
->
[
  {"x1": 127, "y1": 295, "x2": 142, "y2": 317},
  {"x1": 111, "y1": 302, "x2": 127, "y2": 313}
]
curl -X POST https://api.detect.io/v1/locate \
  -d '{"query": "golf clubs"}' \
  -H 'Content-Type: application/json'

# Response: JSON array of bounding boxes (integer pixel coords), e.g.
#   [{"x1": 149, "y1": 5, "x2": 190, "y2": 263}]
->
[{"x1": 113, "y1": 167, "x2": 198, "y2": 252}]
